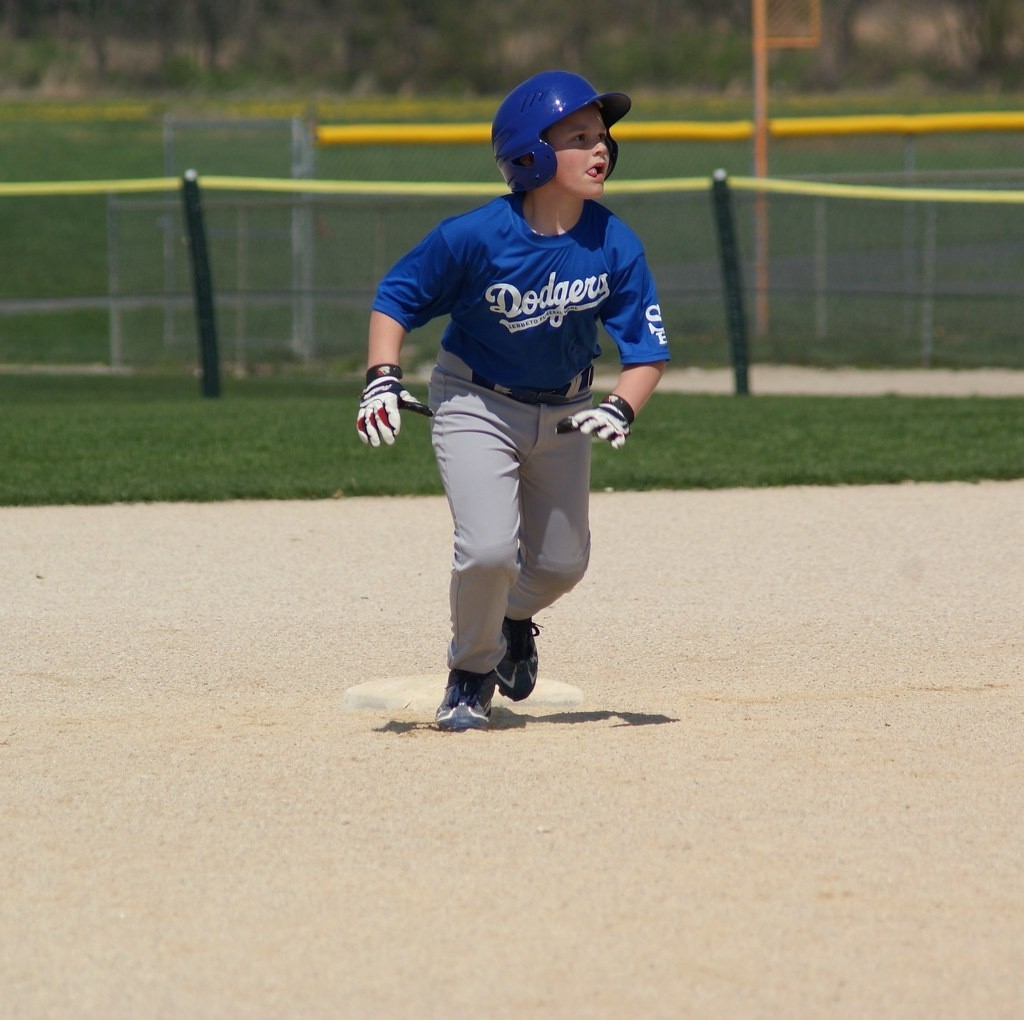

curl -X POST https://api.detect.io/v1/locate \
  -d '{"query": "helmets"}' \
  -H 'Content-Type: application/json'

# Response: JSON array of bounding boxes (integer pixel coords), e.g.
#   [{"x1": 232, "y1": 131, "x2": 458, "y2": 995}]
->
[{"x1": 491, "y1": 72, "x2": 632, "y2": 193}]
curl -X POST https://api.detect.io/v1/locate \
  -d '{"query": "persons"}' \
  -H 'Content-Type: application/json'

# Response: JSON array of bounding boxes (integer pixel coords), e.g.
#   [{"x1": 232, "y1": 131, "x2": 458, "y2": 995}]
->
[{"x1": 355, "y1": 70, "x2": 669, "y2": 730}]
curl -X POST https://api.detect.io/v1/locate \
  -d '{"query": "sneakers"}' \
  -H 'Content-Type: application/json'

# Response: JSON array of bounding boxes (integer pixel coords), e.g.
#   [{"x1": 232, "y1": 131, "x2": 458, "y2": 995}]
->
[
  {"x1": 494, "y1": 613, "x2": 544, "y2": 702},
  {"x1": 435, "y1": 668, "x2": 498, "y2": 730}
]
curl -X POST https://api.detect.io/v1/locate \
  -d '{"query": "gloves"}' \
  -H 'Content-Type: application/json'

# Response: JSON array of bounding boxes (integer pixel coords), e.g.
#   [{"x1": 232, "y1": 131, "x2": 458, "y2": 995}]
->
[
  {"x1": 555, "y1": 394, "x2": 635, "y2": 449},
  {"x1": 356, "y1": 363, "x2": 433, "y2": 447}
]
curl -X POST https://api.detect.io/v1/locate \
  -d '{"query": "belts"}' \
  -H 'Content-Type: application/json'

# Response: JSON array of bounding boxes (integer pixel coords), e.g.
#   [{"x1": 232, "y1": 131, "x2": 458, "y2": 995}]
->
[{"x1": 471, "y1": 364, "x2": 594, "y2": 405}]
